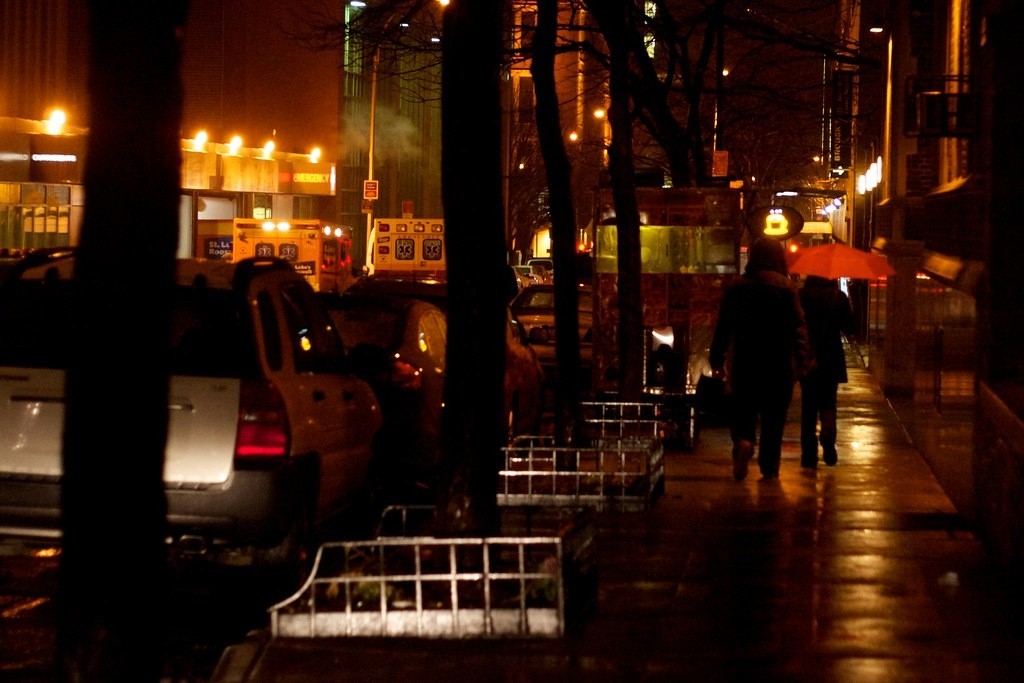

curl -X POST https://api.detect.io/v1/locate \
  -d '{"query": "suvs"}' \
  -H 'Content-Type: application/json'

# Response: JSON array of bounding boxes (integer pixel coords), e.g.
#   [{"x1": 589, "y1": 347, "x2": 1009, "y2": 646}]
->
[{"x1": 0, "y1": 244, "x2": 383, "y2": 623}]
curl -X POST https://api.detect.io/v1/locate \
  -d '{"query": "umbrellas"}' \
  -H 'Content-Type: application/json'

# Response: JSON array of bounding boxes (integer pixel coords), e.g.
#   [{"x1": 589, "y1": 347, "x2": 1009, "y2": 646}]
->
[{"x1": 786, "y1": 243, "x2": 897, "y2": 281}]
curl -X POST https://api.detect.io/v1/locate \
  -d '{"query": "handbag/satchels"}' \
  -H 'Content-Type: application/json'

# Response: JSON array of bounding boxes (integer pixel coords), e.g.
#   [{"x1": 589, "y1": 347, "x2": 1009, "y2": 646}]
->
[{"x1": 696, "y1": 375, "x2": 724, "y2": 411}]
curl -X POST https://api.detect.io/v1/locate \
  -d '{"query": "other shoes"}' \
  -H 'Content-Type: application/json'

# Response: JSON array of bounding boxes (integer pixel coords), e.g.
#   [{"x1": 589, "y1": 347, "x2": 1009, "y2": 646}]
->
[
  {"x1": 732, "y1": 442, "x2": 753, "y2": 480},
  {"x1": 801, "y1": 458, "x2": 818, "y2": 469},
  {"x1": 820, "y1": 430, "x2": 837, "y2": 466},
  {"x1": 758, "y1": 455, "x2": 779, "y2": 479}
]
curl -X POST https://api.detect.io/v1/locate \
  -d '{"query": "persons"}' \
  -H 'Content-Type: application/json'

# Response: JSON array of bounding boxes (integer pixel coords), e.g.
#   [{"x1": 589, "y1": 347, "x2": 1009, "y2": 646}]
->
[
  {"x1": 0, "y1": 247, "x2": 32, "y2": 259},
  {"x1": 708, "y1": 237, "x2": 812, "y2": 480},
  {"x1": 800, "y1": 275, "x2": 854, "y2": 469}
]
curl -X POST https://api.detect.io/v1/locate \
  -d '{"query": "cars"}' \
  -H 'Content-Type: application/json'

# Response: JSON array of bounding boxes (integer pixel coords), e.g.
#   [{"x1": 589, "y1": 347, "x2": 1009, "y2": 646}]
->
[
  {"x1": 512, "y1": 255, "x2": 553, "y2": 288},
  {"x1": 509, "y1": 285, "x2": 595, "y2": 374},
  {"x1": 320, "y1": 270, "x2": 547, "y2": 467}
]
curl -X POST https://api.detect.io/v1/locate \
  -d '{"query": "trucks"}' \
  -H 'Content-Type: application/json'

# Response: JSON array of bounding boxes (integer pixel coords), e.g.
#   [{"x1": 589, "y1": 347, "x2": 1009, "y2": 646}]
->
[
  {"x1": 366, "y1": 216, "x2": 446, "y2": 277},
  {"x1": 234, "y1": 218, "x2": 360, "y2": 297}
]
